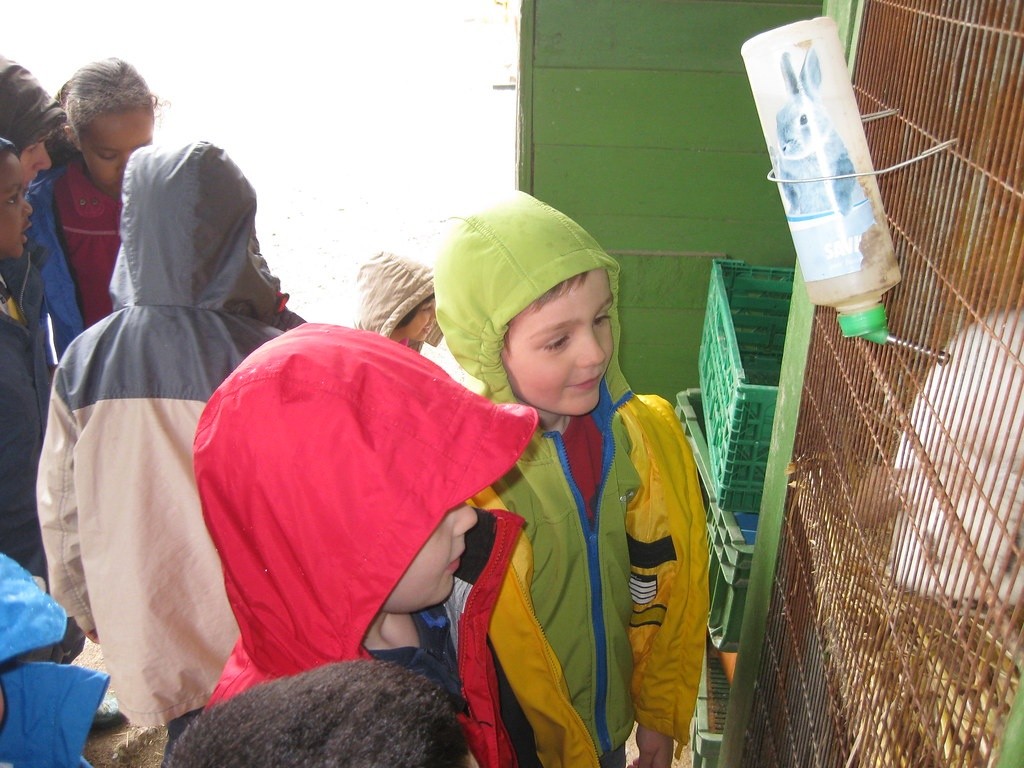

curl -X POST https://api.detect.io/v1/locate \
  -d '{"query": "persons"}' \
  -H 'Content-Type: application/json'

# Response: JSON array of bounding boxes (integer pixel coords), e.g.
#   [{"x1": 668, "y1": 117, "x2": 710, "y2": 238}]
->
[
  {"x1": 349, "y1": 250, "x2": 443, "y2": 354},
  {"x1": 433, "y1": 190, "x2": 710, "y2": 768},
  {"x1": 36, "y1": 139, "x2": 306, "y2": 768},
  {"x1": 167, "y1": 661, "x2": 477, "y2": 768},
  {"x1": 0, "y1": 53, "x2": 67, "y2": 190},
  {"x1": 194, "y1": 322, "x2": 538, "y2": 768},
  {"x1": 0, "y1": 552, "x2": 111, "y2": 768},
  {"x1": 24, "y1": 57, "x2": 158, "y2": 380},
  {"x1": 0, "y1": 139, "x2": 56, "y2": 574}
]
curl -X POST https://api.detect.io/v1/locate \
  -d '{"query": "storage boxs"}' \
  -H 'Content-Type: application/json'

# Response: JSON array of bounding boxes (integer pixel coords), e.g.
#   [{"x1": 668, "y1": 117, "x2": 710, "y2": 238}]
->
[{"x1": 675, "y1": 259, "x2": 795, "y2": 768}]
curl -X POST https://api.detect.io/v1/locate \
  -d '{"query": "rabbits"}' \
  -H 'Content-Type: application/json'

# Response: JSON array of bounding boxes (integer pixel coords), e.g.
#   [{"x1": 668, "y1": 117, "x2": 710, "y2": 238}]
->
[
  {"x1": 768, "y1": 45, "x2": 867, "y2": 218},
  {"x1": 888, "y1": 310, "x2": 1024, "y2": 606}
]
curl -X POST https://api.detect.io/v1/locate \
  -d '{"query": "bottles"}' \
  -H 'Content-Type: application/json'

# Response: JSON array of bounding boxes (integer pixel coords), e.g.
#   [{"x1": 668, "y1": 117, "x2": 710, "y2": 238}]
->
[{"x1": 741, "y1": 17, "x2": 900, "y2": 337}]
